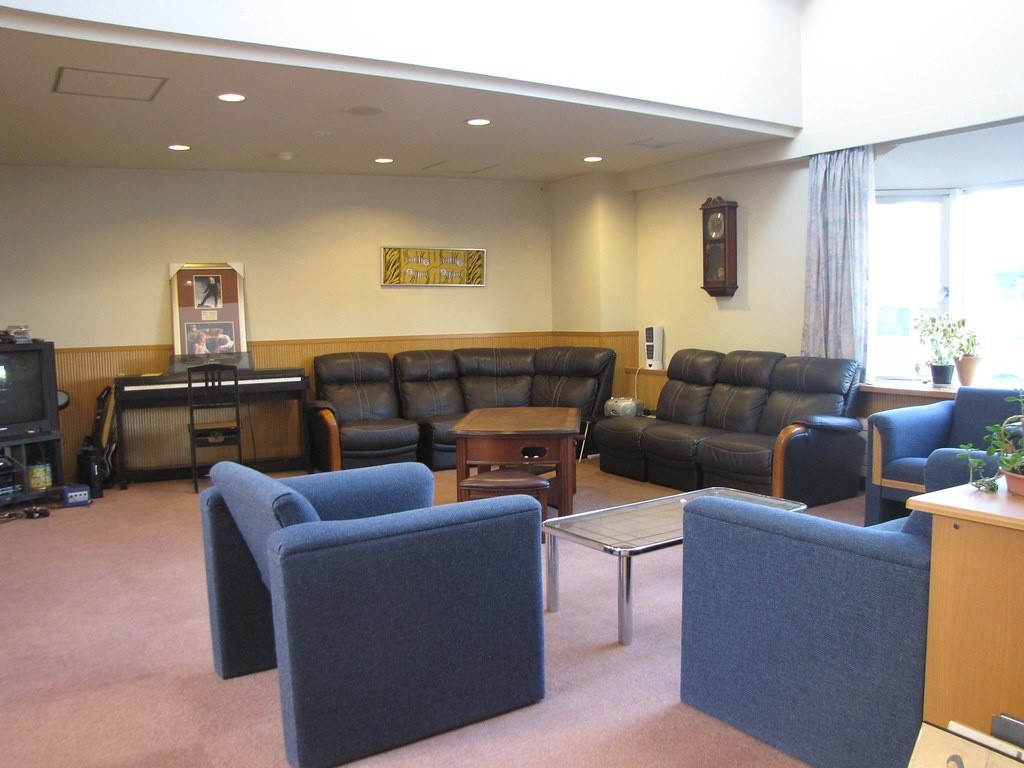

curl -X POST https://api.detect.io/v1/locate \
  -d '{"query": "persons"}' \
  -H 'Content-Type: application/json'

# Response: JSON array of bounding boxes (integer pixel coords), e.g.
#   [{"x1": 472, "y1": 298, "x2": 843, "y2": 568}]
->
[
  {"x1": 188, "y1": 325, "x2": 234, "y2": 358},
  {"x1": 198, "y1": 277, "x2": 220, "y2": 307}
]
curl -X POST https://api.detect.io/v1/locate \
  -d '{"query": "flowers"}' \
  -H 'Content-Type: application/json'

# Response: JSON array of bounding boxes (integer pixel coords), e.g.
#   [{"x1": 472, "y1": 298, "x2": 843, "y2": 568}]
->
[{"x1": 912, "y1": 311, "x2": 980, "y2": 372}]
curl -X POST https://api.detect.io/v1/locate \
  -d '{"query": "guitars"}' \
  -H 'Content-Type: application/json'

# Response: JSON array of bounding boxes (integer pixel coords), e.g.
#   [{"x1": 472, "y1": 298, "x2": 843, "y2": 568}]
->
[{"x1": 74, "y1": 372, "x2": 125, "y2": 488}]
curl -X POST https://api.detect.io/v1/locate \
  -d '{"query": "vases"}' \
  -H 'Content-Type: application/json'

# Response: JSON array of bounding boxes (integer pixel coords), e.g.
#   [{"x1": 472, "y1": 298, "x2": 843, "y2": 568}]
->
[
  {"x1": 954, "y1": 353, "x2": 980, "y2": 386},
  {"x1": 931, "y1": 364, "x2": 954, "y2": 384}
]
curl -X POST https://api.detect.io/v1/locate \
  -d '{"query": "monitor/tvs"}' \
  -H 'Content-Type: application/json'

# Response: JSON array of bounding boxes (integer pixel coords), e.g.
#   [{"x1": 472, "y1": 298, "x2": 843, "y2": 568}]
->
[{"x1": 0, "y1": 341, "x2": 62, "y2": 442}]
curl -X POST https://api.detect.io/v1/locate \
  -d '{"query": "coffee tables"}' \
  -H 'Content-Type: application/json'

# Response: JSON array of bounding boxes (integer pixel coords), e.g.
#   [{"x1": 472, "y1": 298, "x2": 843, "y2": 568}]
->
[{"x1": 542, "y1": 487, "x2": 807, "y2": 645}]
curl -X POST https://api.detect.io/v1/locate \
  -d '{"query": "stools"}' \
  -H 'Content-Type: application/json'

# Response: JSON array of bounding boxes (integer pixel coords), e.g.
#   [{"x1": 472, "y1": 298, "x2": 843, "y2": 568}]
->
[{"x1": 458, "y1": 468, "x2": 550, "y2": 545}]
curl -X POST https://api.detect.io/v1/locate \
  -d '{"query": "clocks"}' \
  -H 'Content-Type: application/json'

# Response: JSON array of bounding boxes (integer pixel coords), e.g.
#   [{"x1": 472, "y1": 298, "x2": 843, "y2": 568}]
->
[{"x1": 699, "y1": 196, "x2": 739, "y2": 297}]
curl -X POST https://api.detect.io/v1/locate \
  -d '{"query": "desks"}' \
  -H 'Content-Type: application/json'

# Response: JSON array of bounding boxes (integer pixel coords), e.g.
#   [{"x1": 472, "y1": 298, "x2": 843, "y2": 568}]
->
[
  {"x1": 453, "y1": 406, "x2": 582, "y2": 519},
  {"x1": 114, "y1": 367, "x2": 310, "y2": 491}
]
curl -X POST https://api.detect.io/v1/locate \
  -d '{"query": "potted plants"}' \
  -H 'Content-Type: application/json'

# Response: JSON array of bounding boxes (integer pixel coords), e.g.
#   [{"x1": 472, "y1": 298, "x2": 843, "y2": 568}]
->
[{"x1": 956, "y1": 388, "x2": 1024, "y2": 496}]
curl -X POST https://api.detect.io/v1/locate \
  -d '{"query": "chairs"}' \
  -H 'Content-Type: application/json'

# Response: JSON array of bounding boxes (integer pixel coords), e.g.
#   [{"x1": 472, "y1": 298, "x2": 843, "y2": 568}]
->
[{"x1": 187, "y1": 364, "x2": 243, "y2": 493}]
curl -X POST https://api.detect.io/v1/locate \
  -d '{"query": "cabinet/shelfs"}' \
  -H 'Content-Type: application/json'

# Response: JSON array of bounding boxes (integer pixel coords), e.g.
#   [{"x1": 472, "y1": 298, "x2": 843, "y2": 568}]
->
[
  {"x1": 906, "y1": 475, "x2": 1024, "y2": 735},
  {"x1": 0, "y1": 432, "x2": 66, "y2": 507}
]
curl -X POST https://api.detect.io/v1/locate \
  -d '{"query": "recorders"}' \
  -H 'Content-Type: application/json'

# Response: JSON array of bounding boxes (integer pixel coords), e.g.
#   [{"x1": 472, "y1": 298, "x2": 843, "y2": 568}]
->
[{"x1": 604, "y1": 396, "x2": 645, "y2": 419}]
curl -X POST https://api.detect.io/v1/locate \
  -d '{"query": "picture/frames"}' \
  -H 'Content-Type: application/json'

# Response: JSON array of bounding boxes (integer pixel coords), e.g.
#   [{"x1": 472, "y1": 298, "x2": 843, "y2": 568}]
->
[
  {"x1": 380, "y1": 246, "x2": 486, "y2": 288},
  {"x1": 169, "y1": 262, "x2": 251, "y2": 374}
]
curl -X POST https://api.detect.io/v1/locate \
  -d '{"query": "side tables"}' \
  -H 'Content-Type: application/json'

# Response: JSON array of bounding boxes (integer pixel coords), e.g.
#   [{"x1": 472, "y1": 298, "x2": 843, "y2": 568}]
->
[{"x1": 587, "y1": 412, "x2": 642, "y2": 454}]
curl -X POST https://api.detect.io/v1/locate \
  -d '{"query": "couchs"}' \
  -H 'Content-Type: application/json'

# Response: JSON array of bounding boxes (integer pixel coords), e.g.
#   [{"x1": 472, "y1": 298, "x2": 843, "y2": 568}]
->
[
  {"x1": 864, "y1": 387, "x2": 1024, "y2": 526},
  {"x1": 306, "y1": 347, "x2": 616, "y2": 473},
  {"x1": 200, "y1": 461, "x2": 545, "y2": 768},
  {"x1": 594, "y1": 348, "x2": 866, "y2": 507},
  {"x1": 680, "y1": 448, "x2": 1017, "y2": 768}
]
